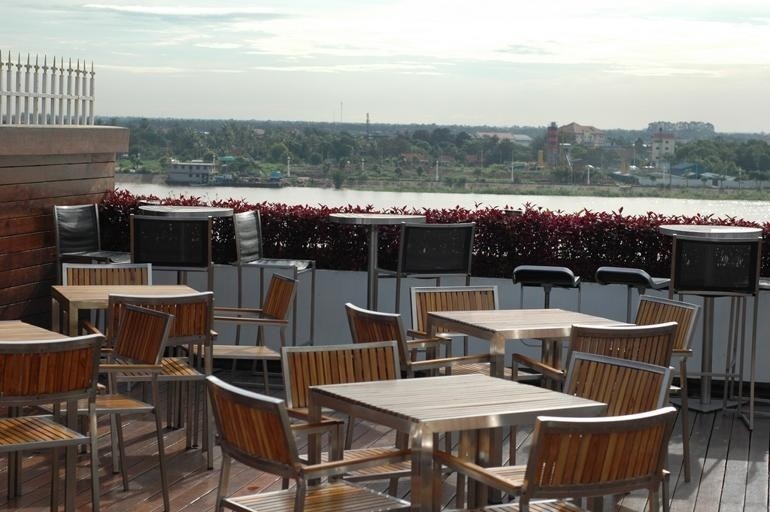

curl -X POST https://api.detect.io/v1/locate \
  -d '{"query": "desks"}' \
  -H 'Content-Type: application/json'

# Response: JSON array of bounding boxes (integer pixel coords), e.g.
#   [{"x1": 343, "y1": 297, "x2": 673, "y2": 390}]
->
[
  {"x1": 0, "y1": 319, "x2": 69, "y2": 354},
  {"x1": 51, "y1": 284, "x2": 204, "y2": 337},
  {"x1": 656, "y1": 223, "x2": 766, "y2": 414},
  {"x1": 328, "y1": 211, "x2": 425, "y2": 310},
  {"x1": 308, "y1": 369, "x2": 606, "y2": 510},
  {"x1": 425, "y1": 308, "x2": 637, "y2": 389},
  {"x1": 138, "y1": 204, "x2": 235, "y2": 287}
]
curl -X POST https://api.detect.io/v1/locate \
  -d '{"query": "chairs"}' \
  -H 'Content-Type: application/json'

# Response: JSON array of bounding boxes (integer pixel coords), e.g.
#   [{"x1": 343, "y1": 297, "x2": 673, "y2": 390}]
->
[
  {"x1": 667, "y1": 232, "x2": 763, "y2": 431},
  {"x1": 60, "y1": 261, "x2": 153, "y2": 286},
  {"x1": 54, "y1": 203, "x2": 129, "y2": 263},
  {"x1": 633, "y1": 293, "x2": 701, "y2": 485},
  {"x1": 3, "y1": 336, "x2": 103, "y2": 512},
  {"x1": 373, "y1": 220, "x2": 476, "y2": 358},
  {"x1": 279, "y1": 339, "x2": 454, "y2": 497},
  {"x1": 86, "y1": 292, "x2": 217, "y2": 468},
  {"x1": 201, "y1": 375, "x2": 414, "y2": 510},
  {"x1": 133, "y1": 216, "x2": 214, "y2": 430},
  {"x1": 345, "y1": 299, "x2": 492, "y2": 470},
  {"x1": 47, "y1": 304, "x2": 174, "y2": 510},
  {"x1": 595, "y1": 265, "x2": 672, "y2": 322},
  {"x1": 511, "y1": 265, "x2": 584, "y2": 391},
  {"x1": 561, "y1": 351, "x2": 673, "y2": 511},
  {"x1": 231, "y1": 211, "x2": 316, "y2": 393},
  {"x1": 433, "y1": 403, "x2": 677, "y2": 511},
  {"x1": 411, "y1": 286, "x2": 542, "y2": 381}
]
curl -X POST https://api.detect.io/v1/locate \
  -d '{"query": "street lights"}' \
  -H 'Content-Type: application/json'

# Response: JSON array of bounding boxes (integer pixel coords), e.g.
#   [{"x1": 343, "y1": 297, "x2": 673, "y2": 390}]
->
[
  {"x1": 585, "y1": 164, "x2": 592, "y2": 185},
  {"x1": 135, "y1": 152, "x2": 142, "y2": 168},
  {"x1": 361, "y1": 158, "x2": 366, "y2": 173},
  {"x1": 435, "y1": 159, "x2": 440, "y2": 182},
  {"x1": 287, "y1": 156, "x2": 291, "y2": 177}
]
